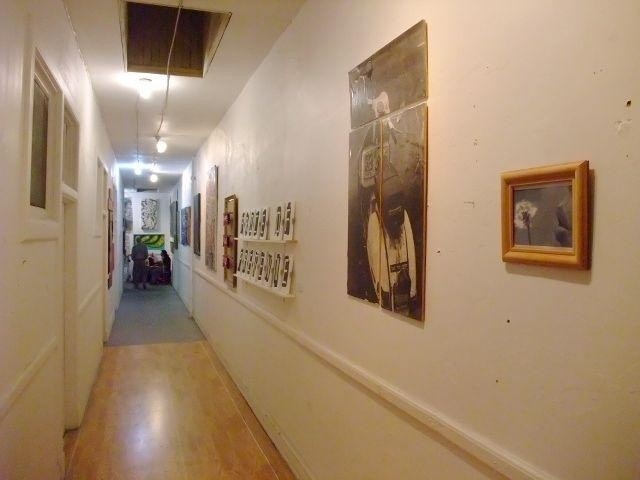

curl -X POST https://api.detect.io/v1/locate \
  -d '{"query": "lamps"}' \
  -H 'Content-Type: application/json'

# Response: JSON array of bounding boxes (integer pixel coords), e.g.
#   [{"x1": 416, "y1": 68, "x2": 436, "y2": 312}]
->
[{"x1": 154, "y1": 135, "x2": 168, "y2": 153}]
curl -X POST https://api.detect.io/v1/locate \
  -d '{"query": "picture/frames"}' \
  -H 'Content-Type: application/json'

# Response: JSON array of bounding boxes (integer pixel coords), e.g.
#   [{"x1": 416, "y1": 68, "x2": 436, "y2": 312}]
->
[
  {"x1": 498, "y1": 160, "x2": 592, "y2": 270},
  {"x1": 237, "y1": 201, "x2": 295, "y2": 296},
  {"x1": 223, "y1": 194, "x2": 238, "y2": 288}
]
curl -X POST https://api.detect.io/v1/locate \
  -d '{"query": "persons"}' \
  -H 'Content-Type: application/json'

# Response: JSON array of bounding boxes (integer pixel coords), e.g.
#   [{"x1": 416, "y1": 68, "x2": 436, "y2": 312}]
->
[
  {"x1": 132, "y1": 236, "x2": 148, "y2": 289},
  {"x1": 161, "y1": 250, "x2": 171, "y2": 274}
]
